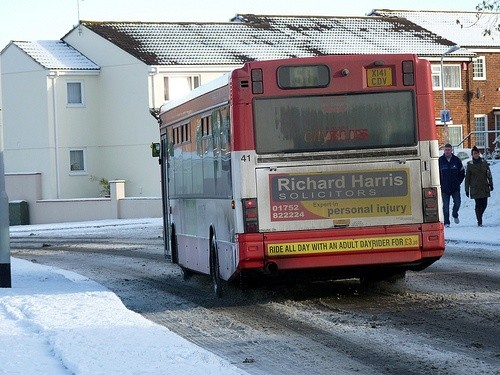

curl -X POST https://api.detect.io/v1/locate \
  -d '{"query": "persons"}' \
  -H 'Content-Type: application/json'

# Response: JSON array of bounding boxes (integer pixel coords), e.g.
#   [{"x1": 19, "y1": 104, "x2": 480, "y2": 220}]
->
[
  {"x1": 438, "y1": 143, "x2": 465, "y2": 227},
  {"x1": 464, "y1": 146, "x2": 494, "y2": 227}
]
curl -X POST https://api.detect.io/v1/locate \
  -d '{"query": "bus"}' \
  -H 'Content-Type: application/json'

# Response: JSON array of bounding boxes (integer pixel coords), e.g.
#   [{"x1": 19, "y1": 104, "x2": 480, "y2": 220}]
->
[{"x1": 149, "y1": 52, "x2": 446, "y2": 299}]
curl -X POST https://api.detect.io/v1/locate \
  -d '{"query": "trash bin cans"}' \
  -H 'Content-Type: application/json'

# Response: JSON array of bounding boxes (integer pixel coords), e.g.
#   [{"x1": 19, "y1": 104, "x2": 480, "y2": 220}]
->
[{"x1": 8, "y1": 200, "x2": 30, "y2": 225}]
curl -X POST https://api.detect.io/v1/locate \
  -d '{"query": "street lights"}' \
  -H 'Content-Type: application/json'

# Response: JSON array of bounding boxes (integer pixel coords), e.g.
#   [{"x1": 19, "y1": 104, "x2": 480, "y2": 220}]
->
[{"x1": 440, "y1": 45, "x2": 461, "y2": 147}]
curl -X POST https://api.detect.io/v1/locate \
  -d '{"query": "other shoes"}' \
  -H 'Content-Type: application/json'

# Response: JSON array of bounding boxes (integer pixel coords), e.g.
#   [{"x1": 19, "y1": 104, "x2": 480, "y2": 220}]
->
[
  {"x1": 445, "y1": 224, "x2": 450, "y2": 228},
  {"x1": 478, "y1": 224, "x2": 483, "y2": 227},
  {"x1": 454, "y1": 218, "x2": 459, "y2": 224}
]
